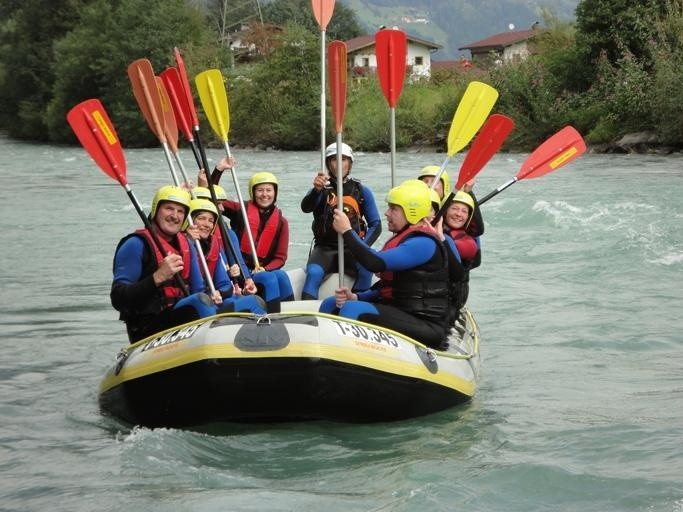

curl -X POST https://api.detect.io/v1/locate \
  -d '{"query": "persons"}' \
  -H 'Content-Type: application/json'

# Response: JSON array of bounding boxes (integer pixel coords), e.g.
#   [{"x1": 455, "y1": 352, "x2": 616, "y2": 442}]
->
[
  {"x1": 109, "y1": 185, "x2": 268, "y2": 344},
  {"x1": 319, "y1": 183, "x2": 452, "y2": 349},
  {"x1": 209, "y1": 154, "x2": 289, "y2": 274},
  {"x1": 443, "y1": 188, "x2": 478, "y2": 262},
  {"x1": 417, "y1": 164, "x2": 486, "y2": 237},
  {"x1": 178, "y1": 177, "x2": 243, "y2": 305},
  {"x1": 299, "y1": 140, "x2": 383, "y2": 301},
  {"x1": 182, "y1": 198, "x2": 269, "y2": 314},
  {"x1": 197, "y1": 167, "x2": 296, "y2": 313},
  {"x1": 423, "y1": 188, "x2": 463, "y2": 327}
]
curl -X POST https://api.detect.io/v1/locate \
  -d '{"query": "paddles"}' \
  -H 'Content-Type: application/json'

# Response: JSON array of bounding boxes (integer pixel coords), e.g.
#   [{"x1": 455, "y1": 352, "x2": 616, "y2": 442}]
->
[
  {"x1": 66, "y1": 97, "x2": 190, "y2": 297},
  {"x1": 312, "y1": 0, "x2": 336, "y2": 175},
  {"x1": 430, "y1": 80, "x2": 499, "y2": 190},
  {"x1": 175, "y1": 47, "x2": 240, "y2": 286},
  {"x1": 151, "y1": 66, "x2": 232, "y2": 266},
  {"x1": 161, "y1": 67, "x2": 247, "y2": 283},
  {"x1": 327, "y1": 40, "x2": 348, "y2": 290},
  {"x1": 430, "y1": 114, "x2": 515, "y2": 226},
  {"x1": 477, "y1": 126, "x2": 587, "y2": 205},
  {"x1": 374, "y1": 30, "x2": 408, "y2": 189},
  {"x1": 128, "y1": 59, "x2": 215, "y2": 297},
  {"x1": 195, "y1": 69, "x2": 259, "y2": 267}
]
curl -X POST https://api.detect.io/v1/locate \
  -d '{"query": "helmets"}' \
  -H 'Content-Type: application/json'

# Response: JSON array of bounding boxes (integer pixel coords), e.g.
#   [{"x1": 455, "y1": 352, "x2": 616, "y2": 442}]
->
[
  {"x1": 248, "y1": 172, "x2": 279, "y2": 204},
  {"x1": 190, "y1": 186, "x2": 213, "y2": 199},
  {"x1": 209, "y1": 184, "x2": 228, "y2": 200},
  {"x1": 149, "y1": 185, "x2": 193, "y2": 234},
  {"x1": 325, "y1": 141, "x2": 354, "y2": 165},
  {"x1": 181, "y1": 198, "x2": 220, "y2": 237},
  {"x1": 452, "y1": 191, "x2": 476, "y2": 233},
  {"x1": 416, "y1": 165, "x2": 449, "y2": 201},
  {"x1": 385, "y1": 179, "x2": 431, "y2": 226},
  {"x1": 427, "y1": 189, "x2": 442, "y2": 210}
]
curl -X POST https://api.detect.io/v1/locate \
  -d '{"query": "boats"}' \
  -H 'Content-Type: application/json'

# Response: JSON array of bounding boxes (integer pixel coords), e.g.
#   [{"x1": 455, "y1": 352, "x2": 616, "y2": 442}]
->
[{"x1": 96, "y1": 266, "x2": 478, "y2": 424}]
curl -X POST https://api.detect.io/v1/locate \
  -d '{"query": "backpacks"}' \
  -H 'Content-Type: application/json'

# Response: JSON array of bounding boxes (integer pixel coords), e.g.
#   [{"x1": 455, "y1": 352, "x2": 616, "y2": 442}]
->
[{"x1": 316, "y1": 193, "x2": 367, "y2": 251}]
